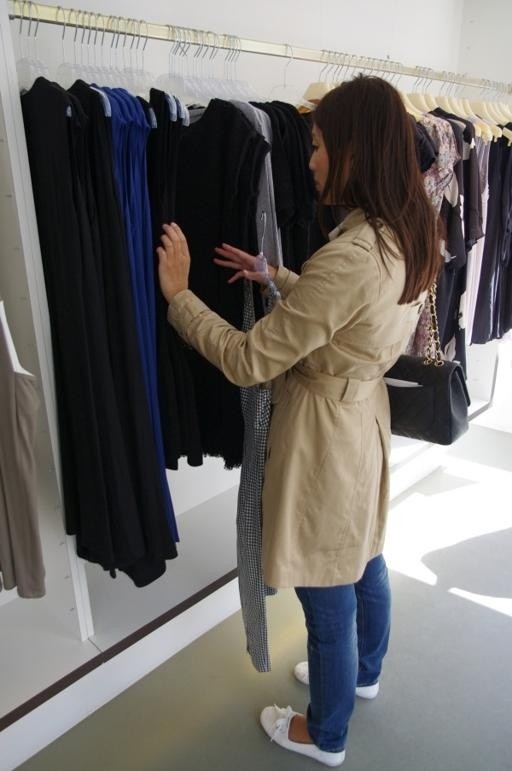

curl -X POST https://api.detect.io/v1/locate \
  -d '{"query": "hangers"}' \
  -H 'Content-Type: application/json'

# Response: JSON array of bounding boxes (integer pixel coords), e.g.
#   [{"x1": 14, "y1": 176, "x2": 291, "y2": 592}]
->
[
  {"x1": 242, "y1": 209, "x2": 283, "y2": 302},
  {"x1": 0, "y1": 298, "x2": 39, "y2": 392}
]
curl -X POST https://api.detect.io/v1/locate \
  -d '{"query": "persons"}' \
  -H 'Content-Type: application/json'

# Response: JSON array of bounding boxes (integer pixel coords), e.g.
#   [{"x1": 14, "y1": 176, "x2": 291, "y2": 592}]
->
[{"x1": 157, "y1": 75, "x2": 443, "y2": 766}]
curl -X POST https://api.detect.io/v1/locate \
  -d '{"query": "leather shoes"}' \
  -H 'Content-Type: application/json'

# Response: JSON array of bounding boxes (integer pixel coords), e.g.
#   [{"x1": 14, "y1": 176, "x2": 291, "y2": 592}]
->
[
  {"x1": 292, "y1": 655, "x2": 381, "y2": 700},
  {"x1": 258, "y1": 705, "x2": 347, "y2": 767}
]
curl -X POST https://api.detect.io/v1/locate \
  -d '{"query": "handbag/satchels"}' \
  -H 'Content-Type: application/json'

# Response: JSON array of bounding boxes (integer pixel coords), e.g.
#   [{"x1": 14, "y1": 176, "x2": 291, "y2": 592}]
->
[{"x1": 383, "y1": 353, "x2": 470, "y2": 447}]
